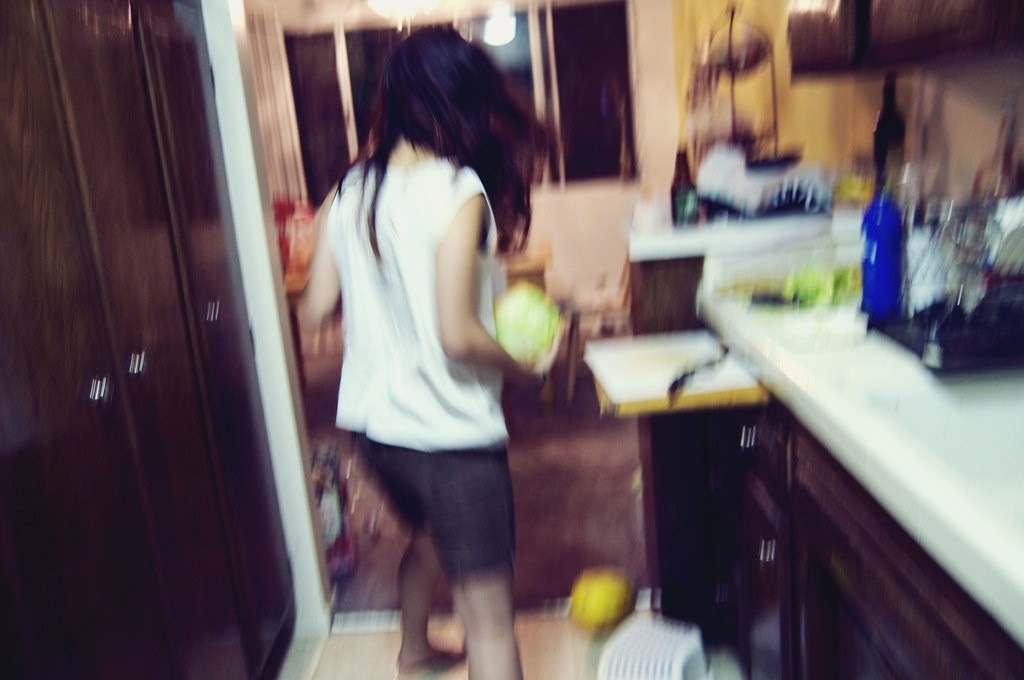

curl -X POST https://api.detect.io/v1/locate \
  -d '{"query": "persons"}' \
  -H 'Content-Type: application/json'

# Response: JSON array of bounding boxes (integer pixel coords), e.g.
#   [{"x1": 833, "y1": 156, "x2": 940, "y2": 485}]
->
[{"x1": 302, "y1": 24, "x2": 563, "y2": 679}]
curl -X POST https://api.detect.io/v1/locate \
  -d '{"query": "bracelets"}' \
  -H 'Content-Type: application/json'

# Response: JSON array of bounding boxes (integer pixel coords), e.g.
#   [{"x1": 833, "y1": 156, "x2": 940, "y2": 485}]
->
[{"x1": 535, "y1": 371, "x2": 549, "y2": 384}]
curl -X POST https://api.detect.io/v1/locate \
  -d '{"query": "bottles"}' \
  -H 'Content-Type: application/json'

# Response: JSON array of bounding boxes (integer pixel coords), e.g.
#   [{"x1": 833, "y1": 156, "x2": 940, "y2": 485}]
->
[
  {"x1": 861, "y1": 190, "x2": 900, "y2": 317},
  {"x1": 873, "y1": 68, "x2": 905, "y2": 189},
  {"x1": 672, "y1": 147, "x2": 699, "y2": 228}
]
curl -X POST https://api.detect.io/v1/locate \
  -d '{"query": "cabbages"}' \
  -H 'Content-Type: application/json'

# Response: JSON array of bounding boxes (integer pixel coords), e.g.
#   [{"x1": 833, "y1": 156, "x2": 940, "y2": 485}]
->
[{"x1": 493, "y1": 280, "x2": 562, "y2": 367}]
